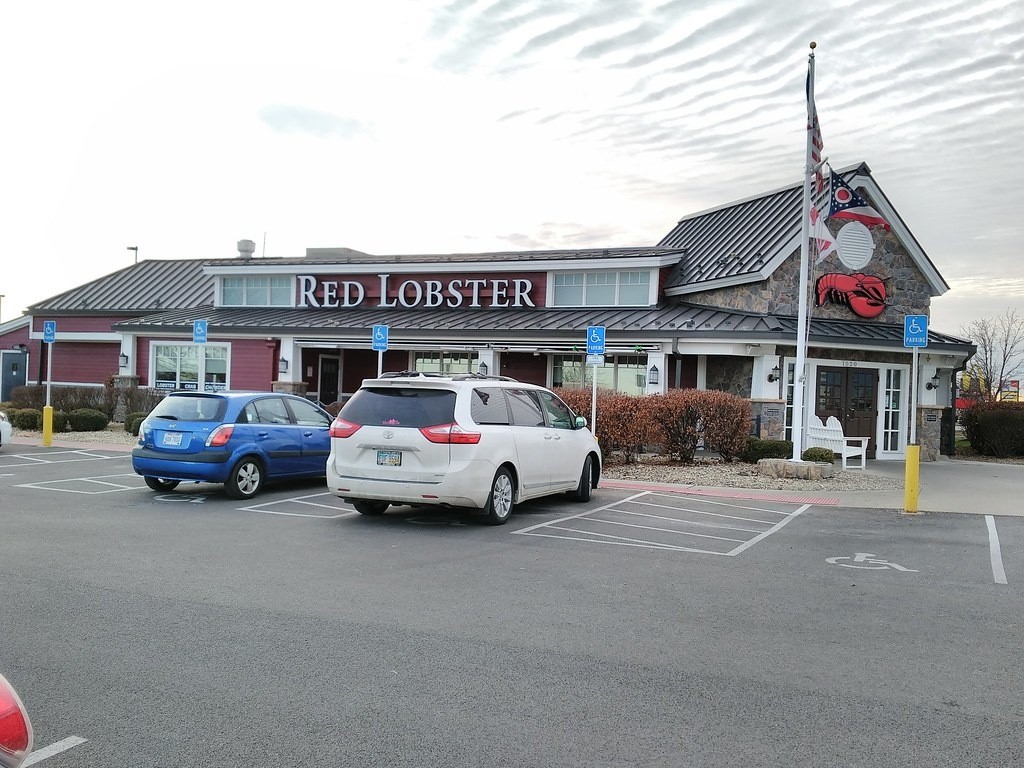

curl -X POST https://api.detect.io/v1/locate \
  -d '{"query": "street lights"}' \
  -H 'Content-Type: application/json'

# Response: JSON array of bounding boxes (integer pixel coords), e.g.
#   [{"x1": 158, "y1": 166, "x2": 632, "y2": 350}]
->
[{"x1": 0, "y1": 295, "x2": 5, "y2": 324}]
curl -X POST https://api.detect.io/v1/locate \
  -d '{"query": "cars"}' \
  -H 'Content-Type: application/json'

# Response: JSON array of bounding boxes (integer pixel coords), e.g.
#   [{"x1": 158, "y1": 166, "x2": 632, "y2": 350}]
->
[
  {"x1": 133, "y1": 392, "x2": 335, "y2": 500},
  {"x1": 327, "y1": 371, "x2": 602, "y2": 525}
]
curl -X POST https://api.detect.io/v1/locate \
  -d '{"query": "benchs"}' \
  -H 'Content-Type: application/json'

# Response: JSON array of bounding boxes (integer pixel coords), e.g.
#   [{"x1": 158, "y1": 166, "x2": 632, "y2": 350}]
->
[{"x1": 808, "y1": 414, "x2": 871, "y2": 471}]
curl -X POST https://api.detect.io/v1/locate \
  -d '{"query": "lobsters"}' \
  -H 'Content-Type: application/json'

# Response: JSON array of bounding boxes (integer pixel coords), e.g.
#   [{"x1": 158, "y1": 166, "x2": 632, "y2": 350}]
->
[{"x1": 816, "y1": 272, "x2": 894, "y2": 318}]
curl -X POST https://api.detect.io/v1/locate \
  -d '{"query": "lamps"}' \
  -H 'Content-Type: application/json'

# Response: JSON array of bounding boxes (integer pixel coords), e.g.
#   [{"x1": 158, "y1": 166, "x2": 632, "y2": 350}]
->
[
  {"x1": 648, "y1": 364, "x2": 659, "y2": 384},
  {"x1": 118, "y1": 351, "x2": 128, "y2": 367},
  {"x1": 926, "y1": 373, "x2": 940, "y2": 390},
  {"x1": 479, "y1": 361, "x2": 487, "y2": 375},
  {"x1": 277, "y1": 356, "x2": 288, "y2": 373},
  {"x1": 766, "y1": 365, "x2": 781, "y2": 383}
]
